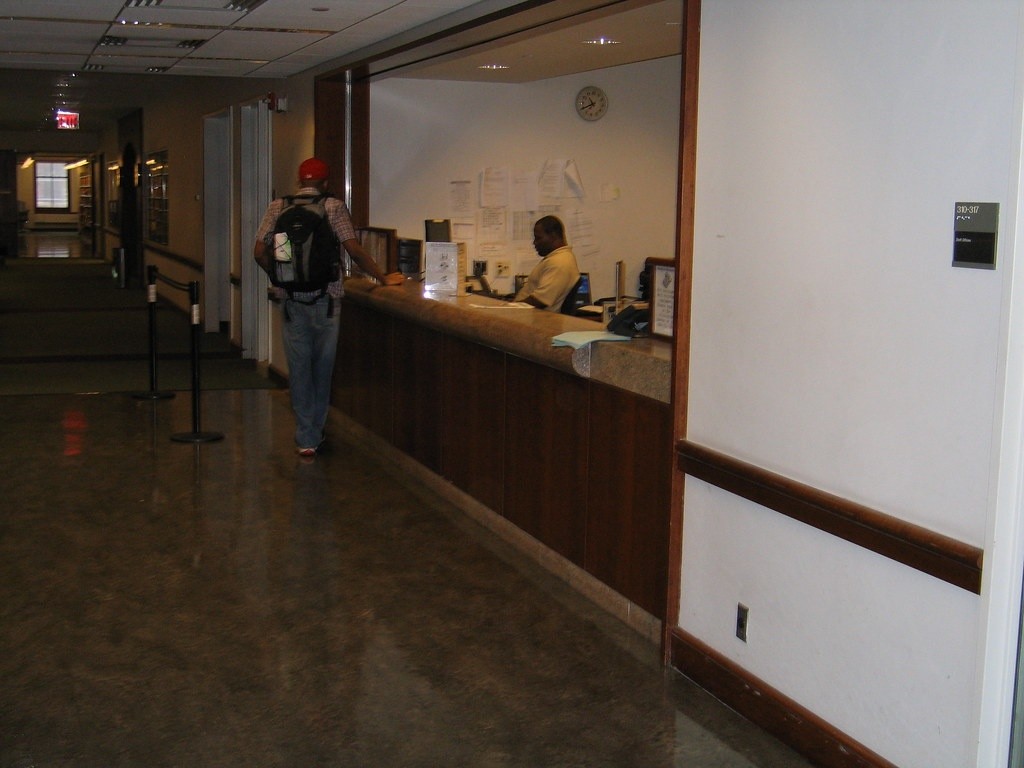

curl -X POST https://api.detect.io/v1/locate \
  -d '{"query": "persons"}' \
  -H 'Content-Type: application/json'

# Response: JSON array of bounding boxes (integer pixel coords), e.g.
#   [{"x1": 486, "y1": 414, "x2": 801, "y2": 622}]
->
[
  {"x1": 254, "y1": 158, "x2": 406, "y2": 456},
  {"x1": 493, "y1": 216, "x2": 581, "y2": 313}
]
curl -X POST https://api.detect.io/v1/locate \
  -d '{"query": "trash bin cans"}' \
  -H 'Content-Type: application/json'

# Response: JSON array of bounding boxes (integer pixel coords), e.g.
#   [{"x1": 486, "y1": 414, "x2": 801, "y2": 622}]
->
[{"x1": 113, "y1": 247, "x2": 130, "y2": 289}]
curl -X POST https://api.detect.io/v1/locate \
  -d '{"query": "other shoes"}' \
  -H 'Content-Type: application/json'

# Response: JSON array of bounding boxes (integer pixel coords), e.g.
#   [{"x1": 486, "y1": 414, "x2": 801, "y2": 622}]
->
[{"x1": 298, "y1": 446, "x2": 316, "y2": 455}]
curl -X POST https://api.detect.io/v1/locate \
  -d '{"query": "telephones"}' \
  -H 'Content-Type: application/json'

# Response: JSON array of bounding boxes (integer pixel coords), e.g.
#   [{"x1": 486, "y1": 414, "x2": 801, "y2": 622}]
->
[{"x1": 606, "y1": 305, "x2": 651, "y2": 336}]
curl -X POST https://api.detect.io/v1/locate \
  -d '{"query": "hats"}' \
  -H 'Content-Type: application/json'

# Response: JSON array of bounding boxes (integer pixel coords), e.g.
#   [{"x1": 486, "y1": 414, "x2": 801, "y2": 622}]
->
[{"x1": 299, "y1": 158, "x2": 329, "y2": 184}]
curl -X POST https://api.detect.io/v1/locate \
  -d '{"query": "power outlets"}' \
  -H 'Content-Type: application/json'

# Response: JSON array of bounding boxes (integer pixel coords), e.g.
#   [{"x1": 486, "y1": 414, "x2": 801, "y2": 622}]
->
[{"x1": 495, "y1": 261, "x2": 511, "y2": 279}]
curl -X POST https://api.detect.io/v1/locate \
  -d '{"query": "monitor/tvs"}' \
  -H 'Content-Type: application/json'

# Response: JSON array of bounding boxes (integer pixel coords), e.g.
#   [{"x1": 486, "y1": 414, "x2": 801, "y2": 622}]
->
[
  {"x1": 573, "y1": 273, "x2": 592, "y2": 309},
  {"x1": 56, "y1": 112, "x2": 79, "y2": 130},
  {"x1": 425, "y1": 219, "x2": 451, "y2": 243}
]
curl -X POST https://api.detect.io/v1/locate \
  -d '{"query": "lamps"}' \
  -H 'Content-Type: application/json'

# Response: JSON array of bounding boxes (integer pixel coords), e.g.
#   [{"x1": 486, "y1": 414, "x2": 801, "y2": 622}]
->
[
  {"x1": 19, "y1": 154, "x2": 35, "y2": 169},
  {"x1": 62, "y1": 157, "x2": 93, "y2": 170},
  {"x1": 107, "y1": 163, "x2": 119, "y2": 172},
  {"x1": 146, "y1": 159, "x2": 157, "y2": 165}
]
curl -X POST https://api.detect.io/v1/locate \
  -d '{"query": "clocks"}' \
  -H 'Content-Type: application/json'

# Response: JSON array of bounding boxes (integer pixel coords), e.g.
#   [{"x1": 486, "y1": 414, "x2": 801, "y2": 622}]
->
[{"x1": 574, "y1": 84, "x2": 610, "y2": 122}]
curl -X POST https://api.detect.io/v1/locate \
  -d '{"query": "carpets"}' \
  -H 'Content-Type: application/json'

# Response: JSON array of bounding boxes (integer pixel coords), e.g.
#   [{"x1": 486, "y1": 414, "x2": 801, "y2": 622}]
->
[{"x1": 0, "y1": 259, "x2": 289, "y2": 392}]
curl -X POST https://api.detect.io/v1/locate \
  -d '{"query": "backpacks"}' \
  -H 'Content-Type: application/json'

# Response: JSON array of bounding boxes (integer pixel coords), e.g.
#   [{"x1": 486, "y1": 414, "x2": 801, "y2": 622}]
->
[{"x1": 266, "y1": 194, "x2": 340, "y2": 290}]
